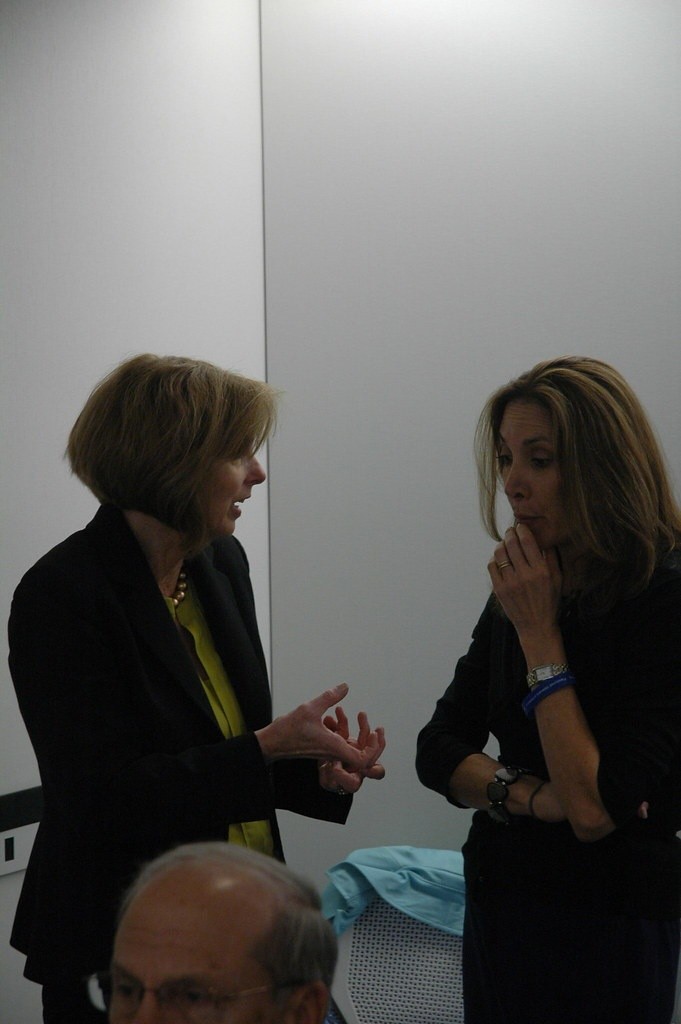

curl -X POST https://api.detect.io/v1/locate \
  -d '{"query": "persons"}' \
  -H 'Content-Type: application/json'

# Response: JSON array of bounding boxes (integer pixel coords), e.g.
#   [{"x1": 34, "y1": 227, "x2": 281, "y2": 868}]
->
[
  {"x1": 416, "y1": 356, "x2": 681, "y2": 1024},
  {"x1": 110, "y1": 841, "x2": 338, "y2": 1024},
  {"x1": 8, "y1": 354, "x2": 386, "y2": 1024}
]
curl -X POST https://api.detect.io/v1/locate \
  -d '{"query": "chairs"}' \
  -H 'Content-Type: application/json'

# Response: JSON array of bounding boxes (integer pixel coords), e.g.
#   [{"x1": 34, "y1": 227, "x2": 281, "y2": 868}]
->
[{"x1": 329, "y1": 846, "x2": 465, "y2": 1024}]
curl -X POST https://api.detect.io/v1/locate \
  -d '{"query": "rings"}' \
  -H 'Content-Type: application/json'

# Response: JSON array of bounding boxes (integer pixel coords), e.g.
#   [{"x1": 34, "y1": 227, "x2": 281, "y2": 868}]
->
[
  {"x1": 336, "y1": 785, "x2": 349, "y2": 795},
  {"x1": 496, "y1": 559, "x2": 512, "y2": 572}
]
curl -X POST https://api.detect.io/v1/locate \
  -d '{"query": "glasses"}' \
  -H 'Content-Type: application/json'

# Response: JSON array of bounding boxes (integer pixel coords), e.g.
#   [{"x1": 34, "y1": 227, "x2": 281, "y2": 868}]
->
[{"x1": 87, "y1": 969, "x2": 278, "y2": 1011}]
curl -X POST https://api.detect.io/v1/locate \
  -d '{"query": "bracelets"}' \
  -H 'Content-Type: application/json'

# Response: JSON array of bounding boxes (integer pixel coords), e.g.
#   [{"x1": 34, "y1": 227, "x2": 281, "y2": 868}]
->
[
  {"x1": 522, "y1": 671, "x2": 578, "y2": 720},
  {"x1": 486, "y1": 764, "x2": 537, "y2": 825},
  {"x1": 529, "y1": 779, "x2": 551, "y2": 823},
  {"x1": 526, "y1": 664, "x2": 572, "y2": 689}
]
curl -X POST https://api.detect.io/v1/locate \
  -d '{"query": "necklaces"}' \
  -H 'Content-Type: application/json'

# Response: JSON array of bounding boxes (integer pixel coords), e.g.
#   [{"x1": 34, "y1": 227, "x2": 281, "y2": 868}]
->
[{"x1": 172, "y1": 572, "x2": 188, "y2": 609}]
